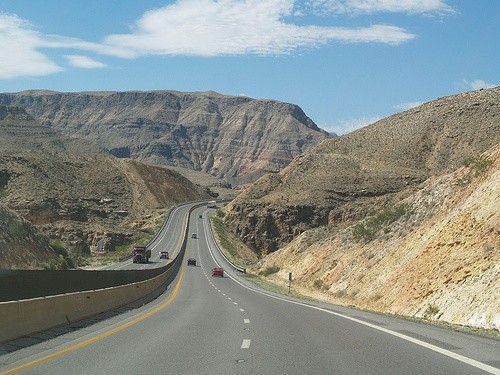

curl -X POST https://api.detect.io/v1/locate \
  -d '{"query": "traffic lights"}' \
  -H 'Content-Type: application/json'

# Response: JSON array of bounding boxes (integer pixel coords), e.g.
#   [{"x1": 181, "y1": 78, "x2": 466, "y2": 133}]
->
[{"x1": 288, "y1": 273, "x2": 292, "y2": 280}]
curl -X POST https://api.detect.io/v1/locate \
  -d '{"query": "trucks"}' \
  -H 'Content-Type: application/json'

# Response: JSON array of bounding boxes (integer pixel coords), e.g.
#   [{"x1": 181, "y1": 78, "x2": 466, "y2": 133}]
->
[{"x1": 131, "y1": 246, "x2": 151, "y2": 264}]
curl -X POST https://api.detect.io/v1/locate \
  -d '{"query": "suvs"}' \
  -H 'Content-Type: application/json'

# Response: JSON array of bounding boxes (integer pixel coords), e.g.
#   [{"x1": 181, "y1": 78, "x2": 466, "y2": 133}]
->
[
  {"x1": 160, "y1": 252, "x2": 168, "y2": 259},
  {"x1": 187, "y1": 258, "x2": 196, "y2": 266},
  {"x1": 211, "y1": 267, "x2": 223, "y2": 277}
]
